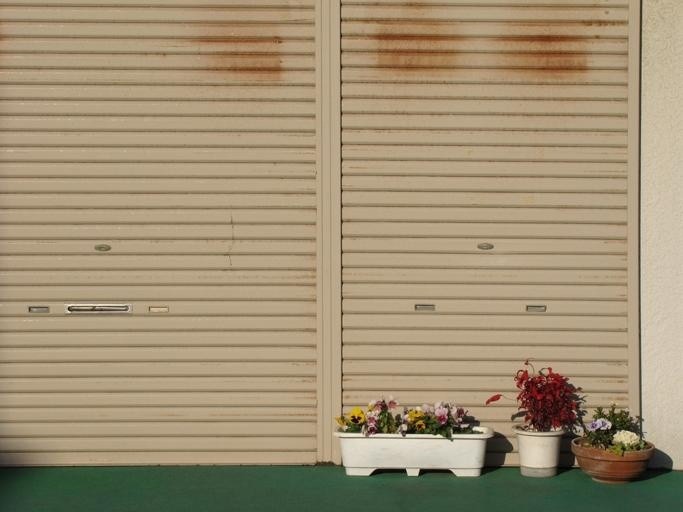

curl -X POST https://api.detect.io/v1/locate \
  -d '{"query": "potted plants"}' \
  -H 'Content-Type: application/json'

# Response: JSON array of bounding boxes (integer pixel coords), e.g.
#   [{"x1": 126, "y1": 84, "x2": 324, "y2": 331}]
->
[{"x1": 484, "y1": 358, "x2": 582, "y2": 477}]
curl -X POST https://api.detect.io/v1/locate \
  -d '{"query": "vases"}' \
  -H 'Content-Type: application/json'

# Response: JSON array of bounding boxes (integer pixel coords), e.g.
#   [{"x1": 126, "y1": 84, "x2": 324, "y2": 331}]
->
[
  {"x1": 334, "y1": 426, "x2": 495, "y2": 478},
  {"x1": 571, "y1": 436, "x2": 656, "y2": 485}
]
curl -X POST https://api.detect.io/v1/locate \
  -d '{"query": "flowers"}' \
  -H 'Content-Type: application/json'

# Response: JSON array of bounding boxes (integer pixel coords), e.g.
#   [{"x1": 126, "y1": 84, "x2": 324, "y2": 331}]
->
[
  {"x1": 584, "y1": 403, "x2": 648, "y2": 457},
  {"x1": 333, "y1": 391, "x2": 468, "y2": 442}
]
